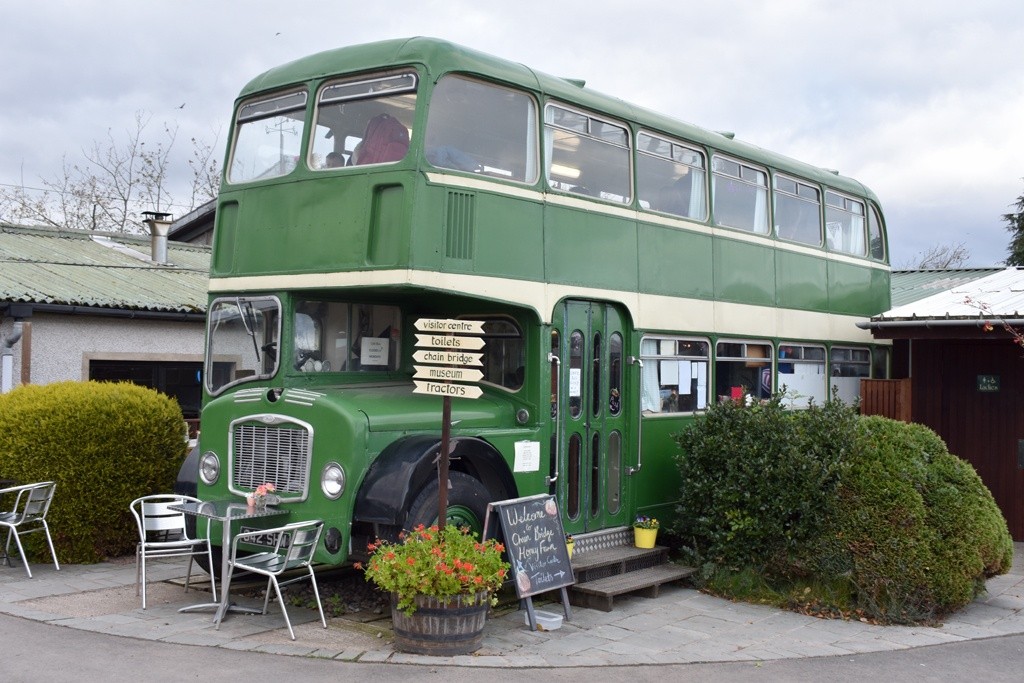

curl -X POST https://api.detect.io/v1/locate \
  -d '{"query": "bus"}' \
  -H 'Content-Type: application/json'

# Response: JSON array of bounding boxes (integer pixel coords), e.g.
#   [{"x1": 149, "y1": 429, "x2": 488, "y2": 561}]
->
[{"x1": 173, "y1": 33, "x2": 894, "y2": 580}]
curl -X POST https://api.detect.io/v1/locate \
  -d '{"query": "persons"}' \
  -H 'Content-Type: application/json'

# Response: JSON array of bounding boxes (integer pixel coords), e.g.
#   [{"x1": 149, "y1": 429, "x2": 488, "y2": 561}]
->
[{"x1": 326, "y1": 152, "x2": 345, "y2": 167}]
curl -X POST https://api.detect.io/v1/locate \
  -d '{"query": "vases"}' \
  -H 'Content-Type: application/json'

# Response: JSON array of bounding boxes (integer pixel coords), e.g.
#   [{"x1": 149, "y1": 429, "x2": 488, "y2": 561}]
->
[
  {"x1": 255, "y1": 496, "x2": 267, "y2": 514},
  {"x1": 391, "y1": 591, "x2": 490, "y2": 657},
  {"x1": 634, "y1": 527, "x2": 659, "y2": 548},
  {"x1": 565, "y1": 542, "x2": 575, "y2": 561}
]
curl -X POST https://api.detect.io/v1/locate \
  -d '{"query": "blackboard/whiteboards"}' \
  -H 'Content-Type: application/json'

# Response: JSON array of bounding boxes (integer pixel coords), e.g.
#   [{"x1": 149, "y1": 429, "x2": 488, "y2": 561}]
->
[{"x1": 482, "y1": 494, "x2": 575, "y2": 599}]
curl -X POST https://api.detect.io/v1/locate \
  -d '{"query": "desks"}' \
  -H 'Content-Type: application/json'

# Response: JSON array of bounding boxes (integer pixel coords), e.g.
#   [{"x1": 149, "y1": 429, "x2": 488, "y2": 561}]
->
[{"x1": 167, "y1": 500, "x2": 291, "y2": 615}]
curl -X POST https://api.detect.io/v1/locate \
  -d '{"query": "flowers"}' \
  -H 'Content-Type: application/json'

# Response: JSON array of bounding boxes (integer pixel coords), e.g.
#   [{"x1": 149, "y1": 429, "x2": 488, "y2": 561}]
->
[
  {"x1": 353, "y1": 523, "x2": 511, "y2": 618},
  {"x1": 631, "y1": 514, "x2": 660, "y2": 529},
  {"x1": 564, "y1": 532, "x2": 574, "y2": 544},
  {"x1": 246, "y1": 483, "x2": 278, "y2": 506}
]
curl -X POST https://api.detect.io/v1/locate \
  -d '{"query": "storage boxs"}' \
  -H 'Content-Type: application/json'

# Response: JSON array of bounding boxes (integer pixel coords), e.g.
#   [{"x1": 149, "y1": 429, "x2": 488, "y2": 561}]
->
[{"x1": 524, "y1": 610, "x2": 563, "y2": 631}]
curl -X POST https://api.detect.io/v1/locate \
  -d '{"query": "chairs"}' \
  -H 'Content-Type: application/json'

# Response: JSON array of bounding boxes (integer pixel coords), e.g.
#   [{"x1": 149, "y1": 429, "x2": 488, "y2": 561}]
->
[
  {"x1": 0, "y1": 480, "x2": 61, "y2": 579},
  {"x1": 215, "y1": 519, "x2": 328, "y2": 642},
  {"x1": 130, "y1": 494, "x2": 217, "y2": 610}
]
